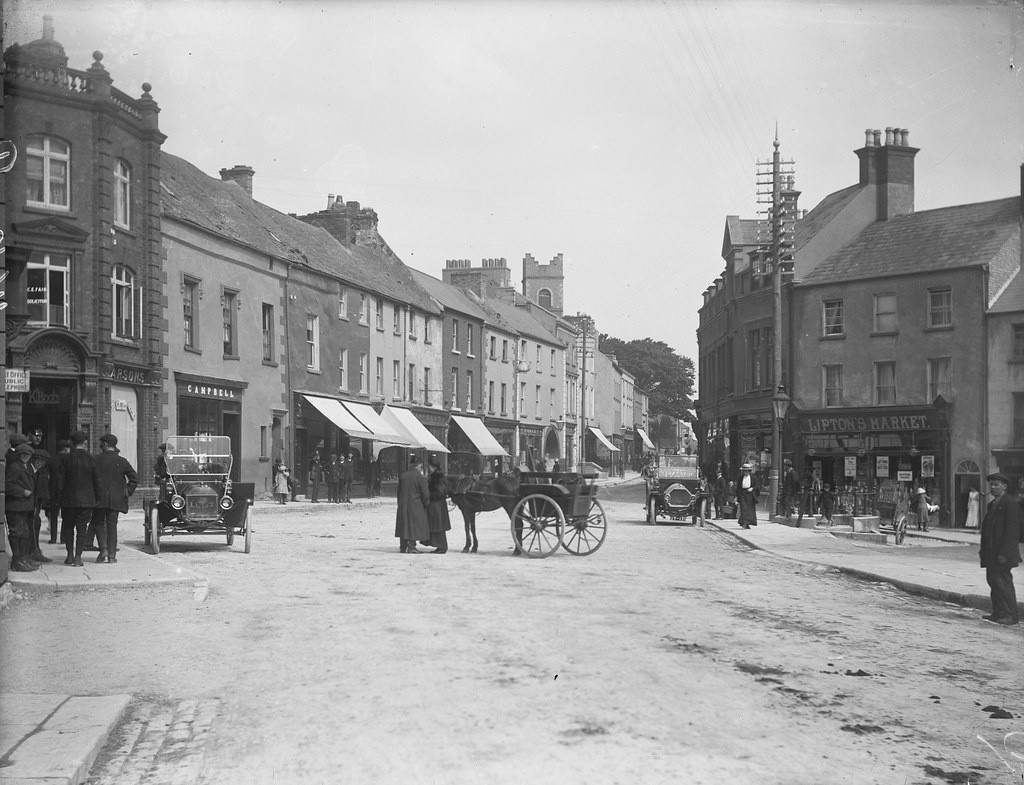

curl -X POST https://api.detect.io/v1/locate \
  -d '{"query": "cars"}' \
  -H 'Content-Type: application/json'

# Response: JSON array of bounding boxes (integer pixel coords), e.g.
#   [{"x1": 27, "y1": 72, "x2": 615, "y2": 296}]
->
[
  {"x1": 645, "y1": 455, "x2": 708, "y2": 528},
  {"x1": 141, "y1": 436, "x2": 256, "y2": 553}
]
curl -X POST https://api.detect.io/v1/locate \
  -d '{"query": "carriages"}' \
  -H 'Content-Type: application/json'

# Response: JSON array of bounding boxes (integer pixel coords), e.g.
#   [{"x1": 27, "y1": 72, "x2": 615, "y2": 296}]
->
[{"x1": 445, "y1": 469, "x2": 608, "y2": 558}]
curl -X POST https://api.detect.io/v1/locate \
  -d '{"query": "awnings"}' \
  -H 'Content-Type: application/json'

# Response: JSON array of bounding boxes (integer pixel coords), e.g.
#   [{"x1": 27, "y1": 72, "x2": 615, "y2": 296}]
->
[
  {"x1": 637, "y1": 428, "x2": 656, "y2": 449},
  {"x1": 589, "y1": 427, "x2": 622, "y2": 452},
  {"x1": 304, "y1": 396, "x2": 511, "y2": 457}
]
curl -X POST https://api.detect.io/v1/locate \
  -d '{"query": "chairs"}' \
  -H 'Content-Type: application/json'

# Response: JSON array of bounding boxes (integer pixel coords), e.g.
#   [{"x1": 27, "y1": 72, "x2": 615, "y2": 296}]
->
[
  {"x1": 170, "y1": 461, "x2": 200, "y2": 474},
  {"x1": 201, "y1": 462, "x2": 229, "y2": 473}
]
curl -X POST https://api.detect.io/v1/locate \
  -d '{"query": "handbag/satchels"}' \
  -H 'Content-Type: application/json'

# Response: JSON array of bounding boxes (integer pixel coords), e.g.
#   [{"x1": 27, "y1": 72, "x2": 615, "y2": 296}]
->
[{"x1": 722, "y1": 505, "x2": 733, "y2": 513}]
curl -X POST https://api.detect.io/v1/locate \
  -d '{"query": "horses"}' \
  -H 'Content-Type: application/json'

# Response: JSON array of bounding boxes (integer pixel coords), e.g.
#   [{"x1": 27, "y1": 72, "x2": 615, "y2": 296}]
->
[{"x1": 444, "y1": 475, "x2": 525, "y2": 556}]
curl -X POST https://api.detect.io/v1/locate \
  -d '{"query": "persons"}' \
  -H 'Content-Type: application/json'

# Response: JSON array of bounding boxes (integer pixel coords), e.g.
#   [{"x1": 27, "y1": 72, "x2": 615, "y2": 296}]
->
[
  {"x1": 965, "y1": 485, "x2": 979, "y2": 527},
  {"x1": 272, "y1": 451, "x2": 451, "y2": 554},
  {"x1": 5, "y1": 424, "x2": 177, "y2": 572},
  {"x1": 979, "y1": 473, "x2": 1022, "y2": 625},
  {"x1": 913, "y1": 487, "x2": 932, "y2": 532},
  {"x1": 700, "y1": 457, "x2": 834, "y2": 529},
  {"x1": 536, "y1": 458, "x2": 560, "y2": 484},
  {"x1": 619, "y1": 444, "x2": 698, "y2": 478}
]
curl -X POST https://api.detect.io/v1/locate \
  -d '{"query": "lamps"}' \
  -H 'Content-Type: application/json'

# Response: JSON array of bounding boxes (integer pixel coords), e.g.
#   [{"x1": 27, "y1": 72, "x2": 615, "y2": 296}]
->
[
  {"x1": 808, "y1": 432, "x2": 815, "y2": 458},
  {"x1": 909, "y1": 430, "x2": 920, "y2": 458},
  {"x1": 855, "y1": 432, "x2": 866, "y2": 458}
]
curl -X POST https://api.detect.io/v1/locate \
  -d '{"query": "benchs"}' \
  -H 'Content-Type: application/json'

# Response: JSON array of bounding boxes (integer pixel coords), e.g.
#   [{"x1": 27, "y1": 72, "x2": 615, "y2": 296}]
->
[{"x1": 520, "y1": 471, "x2": 599, "y2": 497}]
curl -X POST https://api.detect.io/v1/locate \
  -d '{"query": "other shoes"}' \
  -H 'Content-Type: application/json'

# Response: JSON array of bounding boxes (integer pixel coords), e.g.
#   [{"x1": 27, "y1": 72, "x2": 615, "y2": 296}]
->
[
  {"x1": 96, "y1": 555, "x2": 117, "y2": 563},
  {"x1": 430, "y1": 548, "x2": 446, "y2": 553},
  {"x1": 31, "y1": 548, "x2": 52, "y2": 562},
  {"x1": 400, "y1": 549, "x2": 405, "y2": 553},
  {"x1": 10, "y1": 556, "x2": 41, "y2": 571},
  {"x1": 49, "y1": 539, "x2": 65, "y2": 544},
  {"x1": 982, "y1": 610, "x2": 1019, "y2": 625},
  {"x1": 408, "y1": 546, "x2": 423, "y2": 553},
  {"x1": 64, "y1": 557, "x2": 84, "y2": 566},
  {"x1": 83, "y1": 546, "x2": 98, "y2": 550}
]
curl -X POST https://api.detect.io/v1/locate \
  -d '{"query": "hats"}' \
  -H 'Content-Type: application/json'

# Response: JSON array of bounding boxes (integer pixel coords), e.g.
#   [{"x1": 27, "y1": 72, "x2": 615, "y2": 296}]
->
[
  {"x1": 279, "y1": 466, "x2": 288, "y2": 471},
  {"x1": 916, "y1": 487, "x2": 926, "y2": 495},
  {"x1": 824, "y1": 483, "x2": 830, "y2": 488},
  {"x1": 9, "y1": 423, "x2": 121, "y2": 462},
  {"x1": 276, "y1": 457, "x2": 281, "y2": 461},
  {"x1": 410, "y1": 455, "x2": 423, "y2": 464},
  {"x1": 428, "y1": 458, "x2": 441, "y2": 471},
  {"x1": 986, "y1": 473, "x2": 1011, "y2": 486},
  {"x1": 784, "y1": 459, "x2": 792, "y2": 465},
  {"x1": 739, "y1": 464, "x2": 752, "y2": 471},
  {"x1": 158, "y1": 442, "x2": 174, "y2": 450},
  {"x1": 330, "y1": 450, "x2": 354, "y2": 458}
]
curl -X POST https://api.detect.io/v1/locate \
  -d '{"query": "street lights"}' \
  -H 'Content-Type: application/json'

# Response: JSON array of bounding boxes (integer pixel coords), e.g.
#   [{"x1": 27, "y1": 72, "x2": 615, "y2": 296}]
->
[{"x1": 769, "y1": 385, "x2": 791, "y2": 520}]
